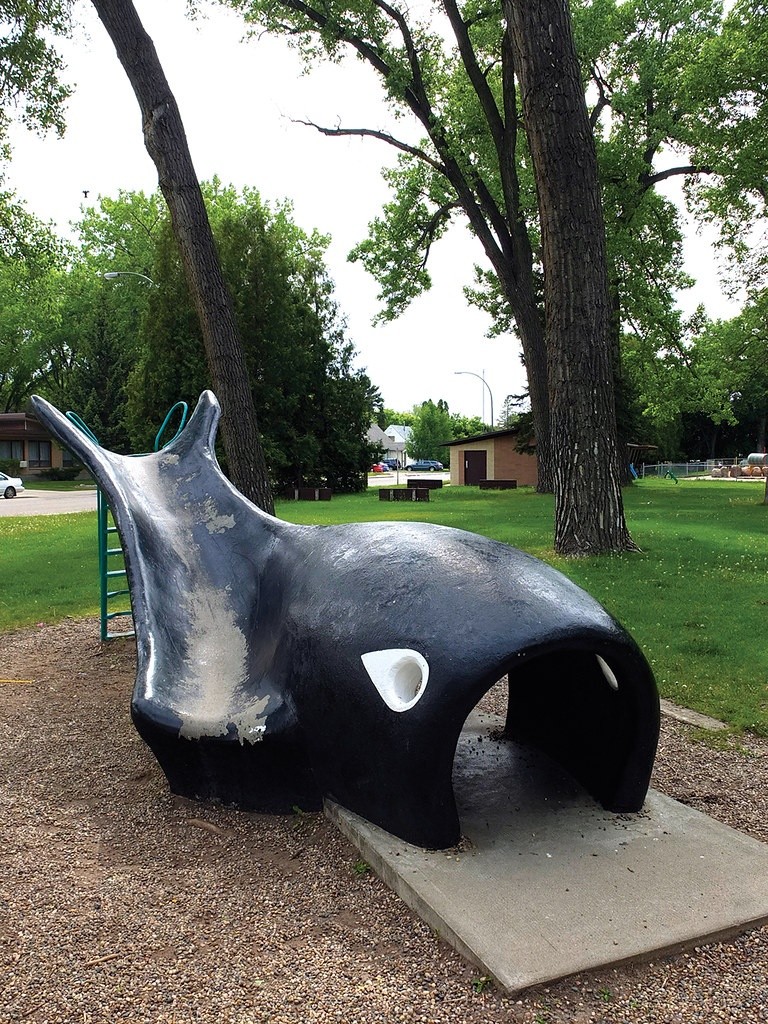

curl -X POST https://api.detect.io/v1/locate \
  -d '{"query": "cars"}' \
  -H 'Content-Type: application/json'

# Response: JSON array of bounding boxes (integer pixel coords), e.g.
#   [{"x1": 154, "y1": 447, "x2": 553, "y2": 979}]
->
[
  {"x1": 373, "y1": 462, "x2": 389, "y2": 472},
  {"x1": 405, "y1": 461, "x2": 443, "y2": 471},
  {"x1": 0, "y1": 472, "x2": 25, "y2": 499}
]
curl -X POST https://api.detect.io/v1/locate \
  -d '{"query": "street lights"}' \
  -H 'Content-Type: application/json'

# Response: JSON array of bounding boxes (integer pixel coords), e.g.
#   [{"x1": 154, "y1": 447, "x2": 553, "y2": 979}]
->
[
  {"x1": 105, "y1": 272, "x2": 160, "y2": 289},
  {"x1": 455, "y1": 371, "x2": 495, "y2": 432}
]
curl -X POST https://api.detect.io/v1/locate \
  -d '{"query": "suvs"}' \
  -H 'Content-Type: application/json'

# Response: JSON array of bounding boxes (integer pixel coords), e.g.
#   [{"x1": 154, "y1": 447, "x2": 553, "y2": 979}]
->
[{"x1": 384, "y1": 458, "x2": 400, "y2": 470}]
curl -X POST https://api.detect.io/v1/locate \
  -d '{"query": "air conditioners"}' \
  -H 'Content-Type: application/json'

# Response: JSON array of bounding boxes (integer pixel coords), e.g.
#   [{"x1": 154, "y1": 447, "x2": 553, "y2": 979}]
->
[{"x1": 20, "y1": 461, "x2": 27, "y2": 468}]
